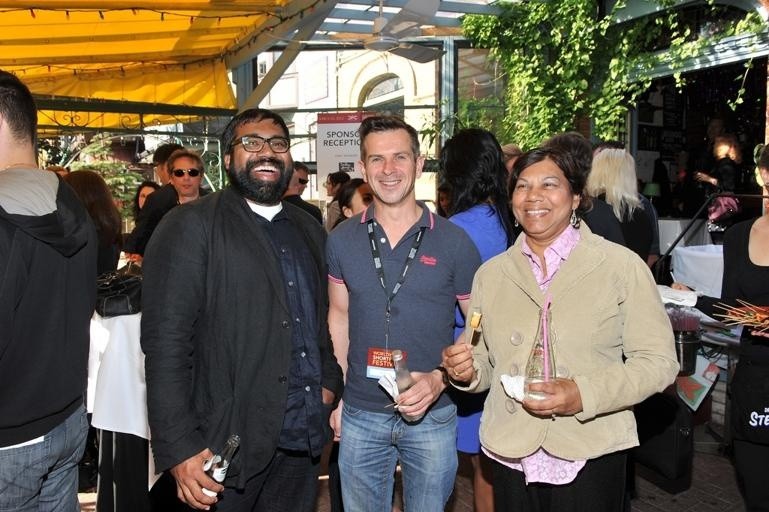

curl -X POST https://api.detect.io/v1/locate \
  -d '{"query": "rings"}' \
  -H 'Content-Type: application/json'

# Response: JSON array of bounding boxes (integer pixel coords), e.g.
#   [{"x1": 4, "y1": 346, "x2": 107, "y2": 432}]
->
[
  {"x1": 454, "y1": 368, "x2": 459, "y2": 376},
  {"x1": 550, "y1": 406, "x2": 558, "y2": 422}
]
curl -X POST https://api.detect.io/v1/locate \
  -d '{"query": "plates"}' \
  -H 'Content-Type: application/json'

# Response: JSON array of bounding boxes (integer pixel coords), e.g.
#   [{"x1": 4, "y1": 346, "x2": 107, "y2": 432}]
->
[{"x1": 726, "y1": 306, "x2": 769, "y2": 317}]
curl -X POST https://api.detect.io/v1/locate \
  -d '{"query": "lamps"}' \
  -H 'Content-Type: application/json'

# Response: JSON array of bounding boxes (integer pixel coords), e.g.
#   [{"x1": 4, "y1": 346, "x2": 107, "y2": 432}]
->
[{"x1": 642, "y1": 183, "x2": 661, "y2": 204}]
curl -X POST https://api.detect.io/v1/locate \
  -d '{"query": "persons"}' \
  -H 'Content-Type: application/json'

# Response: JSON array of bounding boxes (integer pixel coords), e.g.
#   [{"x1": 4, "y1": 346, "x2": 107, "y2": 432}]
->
[
  {"x1": 692, "y1": 133, "x2": 744, "y2": 244},
  {"x1": 438, "y1": 127, "x2": 517, "y2": 512},
  {"x1": 699, "y1": 116, "x2": 725, "y2": 190},
  {"x1": 324, "y1": 115, "x2": 483, "y2": 512},
  {"x1": 140, "y1": 108, "x2": 345, "y2": 511},
  {"x1": 671, "y1": 144, "x2": 769, "y2": 512},
  {"x1": 593, "y1": 139, "x2": 660, "y2": 267},
  {"x1": 0, "y1": 70, "x2": 99, "y2": 511},
  {"x1": 139, "y1": 148, "x2": 204, "y2": 255},
  {"x1": 283, "y1": 168, "x2": 323, "y2": 226},
  {"x1": 332, "y1": 178, "x2": 373, "y2": 230},
  {"x1": 132, "y1": 180, "x2": 161, "y2": 227},
  {"x1": 325, "y1": 171, "x2": 350, "y2": 231},
  {"x1": 502, "y1": 143, "x2": 523, "y2": 193},
  {"x1": 435, "y1": 181, "x2": 450, "y2": 217},
  {"x1": 294, "y1": 161, "x2": 309, "y2": 196},
  {"x1": 63, "y1": 170, "x2": 122, "y2": 493},
  {"x1": 126, "y1": 142, "x2": 209, "y2": 254},
  {"x1": 584, "y1": 148, "x2": 653, "y2": 262},
  {"x1": 546, "y1": 134, "x2": 626, "y2": 245},
  {"x1": 441, "y1": 145, "x2": 682, "y2": 512}
]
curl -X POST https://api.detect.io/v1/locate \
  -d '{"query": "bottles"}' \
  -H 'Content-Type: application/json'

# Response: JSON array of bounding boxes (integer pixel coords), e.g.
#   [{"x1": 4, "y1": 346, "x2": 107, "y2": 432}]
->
[
  {"x1": 524, "y1": 308, "x2": 557, "y2": 402},
  {"x1": 392, "y1": 349, "x2": 425, "y2": 423},
  {"x1": 191, "y1": 434, "x2": 240, "y2": 507}
]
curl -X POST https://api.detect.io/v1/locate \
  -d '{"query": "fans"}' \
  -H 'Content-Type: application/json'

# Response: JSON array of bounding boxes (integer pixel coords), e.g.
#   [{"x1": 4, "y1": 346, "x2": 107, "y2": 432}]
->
[{"x1": 263, "y1": 0, "x2": 448, "y2": 64}]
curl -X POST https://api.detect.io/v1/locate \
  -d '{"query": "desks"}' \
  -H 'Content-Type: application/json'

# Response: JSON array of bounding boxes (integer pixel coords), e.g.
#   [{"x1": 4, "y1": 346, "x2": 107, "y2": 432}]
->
[
  {"x1": 658, "y1": 218, "x2": 712, "y2": 284},
  {"x1": 670, "y1": 244, "x2": 723, "y2": 300}
]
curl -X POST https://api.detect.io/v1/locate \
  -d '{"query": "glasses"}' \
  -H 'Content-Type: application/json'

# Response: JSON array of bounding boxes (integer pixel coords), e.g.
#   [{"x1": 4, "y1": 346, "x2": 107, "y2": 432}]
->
[
  {"x1": 174, "y1": 169, "x2": 199, "y2": 177},
  {"x1": 152, "y1": 162, "x2": 159, "y2": 171},
  {"x1": 299, "y1": 178, "x2": 309, "y2": 184},
  {"x1": 231, "y1": 134, "x2": 290, "y2": 153},
  {"x1": 322, "y1": 182, "x2": 330, "y2": 187}
]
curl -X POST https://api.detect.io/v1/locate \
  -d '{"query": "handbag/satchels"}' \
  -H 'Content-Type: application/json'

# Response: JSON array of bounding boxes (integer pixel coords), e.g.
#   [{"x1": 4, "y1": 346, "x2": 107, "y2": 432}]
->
[{"x1": 96, "y1": 260, "x2": 142, "y2": 317}]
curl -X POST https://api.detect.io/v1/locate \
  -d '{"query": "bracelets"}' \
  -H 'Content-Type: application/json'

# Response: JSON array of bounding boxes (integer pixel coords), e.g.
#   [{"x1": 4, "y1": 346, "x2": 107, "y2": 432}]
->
[{"x1": 436, "y1": 366, "x2": 451, "y2": 386}]
